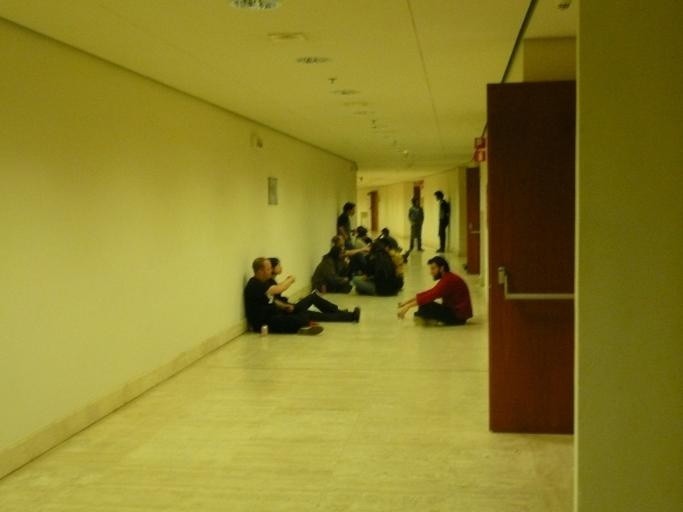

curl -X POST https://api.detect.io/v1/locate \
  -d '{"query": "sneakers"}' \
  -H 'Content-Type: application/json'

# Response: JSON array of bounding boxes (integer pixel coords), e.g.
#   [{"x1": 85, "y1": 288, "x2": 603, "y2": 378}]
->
[{"x1": 298, "y1": 326, "x2": 322, "y2": 335}]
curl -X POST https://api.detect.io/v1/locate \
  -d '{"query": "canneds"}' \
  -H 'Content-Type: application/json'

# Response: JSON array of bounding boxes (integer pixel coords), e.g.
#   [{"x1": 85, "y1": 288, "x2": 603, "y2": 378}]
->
[{"x1": 262, "y1": 325, "x2": 269, "y2": 336}]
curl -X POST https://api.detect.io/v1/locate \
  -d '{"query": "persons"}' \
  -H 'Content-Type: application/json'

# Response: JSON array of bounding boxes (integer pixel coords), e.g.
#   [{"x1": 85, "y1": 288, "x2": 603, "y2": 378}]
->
[
  {"x1": 432, "y1": 191, "x2": 451, "y2": 253},
  {"x1": 308, "y1": 200, "x2": 405, "y2": 296},
  {"x1": 240, "y1": 257, "x2": 323, "y2": 336},
  {"x1": 407, "y1": 196, "x2": 424, "y2": 254},
  {"x1": 269, "y1": 256, "x2": 360, "y2": 324},
  {"x1": 396, "y1": 254, "x2": 473, "y2": 327}
]
